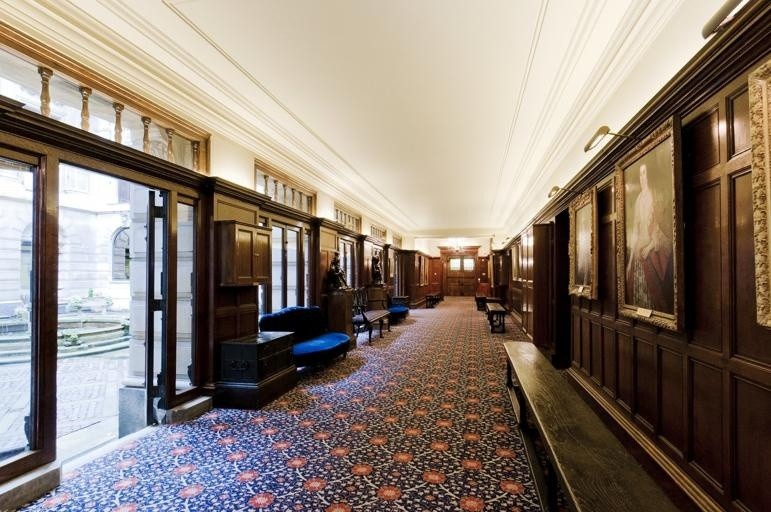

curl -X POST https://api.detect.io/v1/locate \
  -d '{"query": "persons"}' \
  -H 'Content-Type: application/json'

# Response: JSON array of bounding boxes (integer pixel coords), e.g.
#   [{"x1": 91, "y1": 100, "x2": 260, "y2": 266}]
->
[
  {"x1": 625, "y1": 161, "x2": 675, "y2": 316},
  {"x1": 330, "y1": 252, "x2": 353, "y2": 290},
  {"x1": 372, "y1": 250, "x2": 385, "y2": 286}
]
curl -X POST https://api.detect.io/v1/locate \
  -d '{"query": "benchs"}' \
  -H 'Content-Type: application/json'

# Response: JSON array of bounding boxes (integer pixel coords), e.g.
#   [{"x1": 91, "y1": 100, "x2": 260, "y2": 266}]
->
[
  {"x1": 502, "y1": 341, "x2": 684, "y2": 512},
  {"x1": 474, "y1": 290, "x2": 507, "y2": 334},
  {"x1": 424, "y1": 291, "x2": 441, "y2": 308}
]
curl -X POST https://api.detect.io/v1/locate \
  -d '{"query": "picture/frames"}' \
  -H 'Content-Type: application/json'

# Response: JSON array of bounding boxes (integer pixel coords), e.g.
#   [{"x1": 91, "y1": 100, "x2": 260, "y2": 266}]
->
[
  {"x1": 746, "y1": 59, "x2": 770, "y2": 328},
  {"x1": 614, "y1": 114, "x2": 683, "y2": 333},
  {"x1": 566, "y1": 185, "x2": 598, "y2": 302}
]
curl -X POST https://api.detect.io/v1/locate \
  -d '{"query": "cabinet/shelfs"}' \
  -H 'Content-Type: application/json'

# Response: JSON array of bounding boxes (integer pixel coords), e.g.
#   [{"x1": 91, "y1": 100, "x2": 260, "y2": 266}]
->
[
  {"x1": 217, "y1": 220, "x2": 272, "y2": 287},
  {"x1": 220, "y1": 331, "x2": 294, "y2": 384}
]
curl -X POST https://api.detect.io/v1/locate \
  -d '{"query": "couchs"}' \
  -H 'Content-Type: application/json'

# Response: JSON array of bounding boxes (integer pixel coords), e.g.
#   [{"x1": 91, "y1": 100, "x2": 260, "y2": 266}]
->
[{"x1": 258, "y1": 305, "x2": 351, "y2": 375}]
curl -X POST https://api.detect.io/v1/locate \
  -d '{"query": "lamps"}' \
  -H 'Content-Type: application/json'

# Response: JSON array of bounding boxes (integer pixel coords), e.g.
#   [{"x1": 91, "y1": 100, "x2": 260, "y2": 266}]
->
[
  {"x1": 583, "y1": 125, "x2": 636, "y2": 152},
  {"x1": 548, "y1": 185, "x2": 583, "y2": 199},
  {"x1": 701, "y1": 0, "x2": 744, "y2": 39}
]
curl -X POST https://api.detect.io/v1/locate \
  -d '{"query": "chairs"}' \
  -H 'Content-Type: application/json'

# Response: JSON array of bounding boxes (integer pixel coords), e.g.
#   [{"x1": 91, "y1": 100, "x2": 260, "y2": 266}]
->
[{"x1": 344, "y1": 287, "x2": 392, "y2": 347}]
